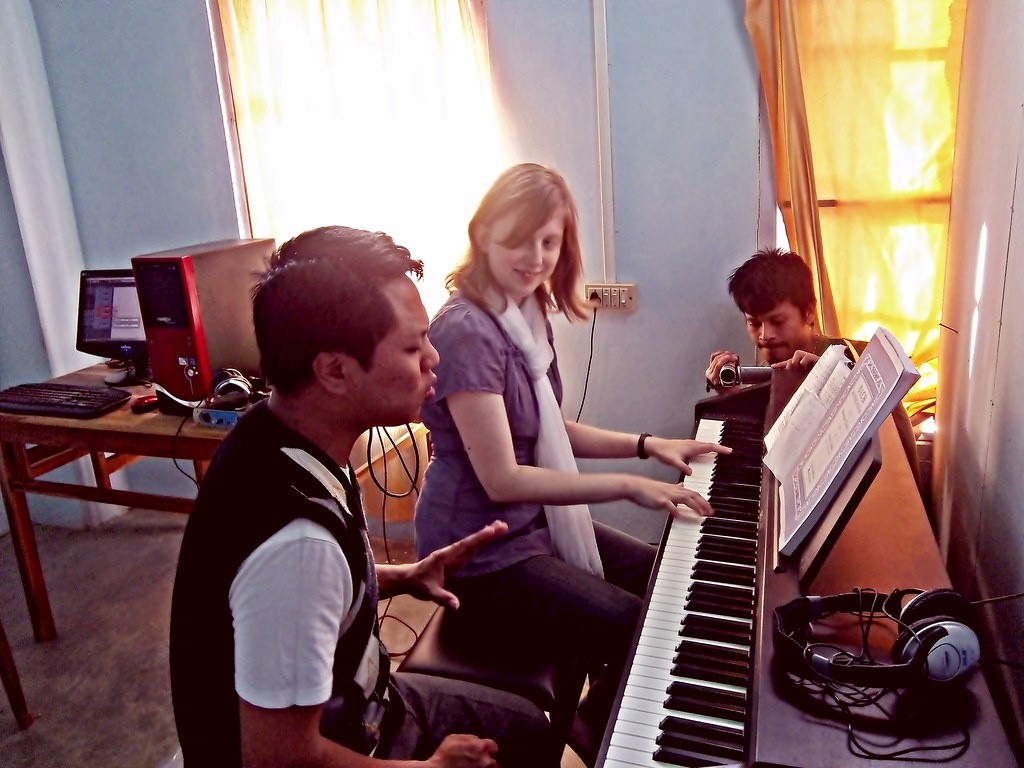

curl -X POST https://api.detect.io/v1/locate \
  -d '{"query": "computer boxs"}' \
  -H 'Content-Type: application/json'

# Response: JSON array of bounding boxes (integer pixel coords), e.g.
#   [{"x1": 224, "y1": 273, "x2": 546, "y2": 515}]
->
[{"x1": 130, "y1": 239, "x2": 278, "y2": 417}]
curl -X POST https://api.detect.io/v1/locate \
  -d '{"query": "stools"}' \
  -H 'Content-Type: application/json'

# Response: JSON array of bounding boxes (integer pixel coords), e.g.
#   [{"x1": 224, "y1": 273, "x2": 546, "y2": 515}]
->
[{"x1": 396, "y1": 602, "x2": 587, "y2": 768}]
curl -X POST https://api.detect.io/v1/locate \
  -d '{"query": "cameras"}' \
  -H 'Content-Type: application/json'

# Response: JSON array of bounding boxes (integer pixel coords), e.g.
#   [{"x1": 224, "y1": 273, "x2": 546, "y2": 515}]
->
[{"x1": 718, "y1": 353, "x2": 772, "y2": 388}]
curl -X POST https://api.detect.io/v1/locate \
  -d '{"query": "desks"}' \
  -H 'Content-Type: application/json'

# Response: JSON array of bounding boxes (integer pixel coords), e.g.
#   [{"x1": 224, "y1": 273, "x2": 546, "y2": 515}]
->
[{"x1": 1, "y1": 361, "x2": 434, "y2": 644}]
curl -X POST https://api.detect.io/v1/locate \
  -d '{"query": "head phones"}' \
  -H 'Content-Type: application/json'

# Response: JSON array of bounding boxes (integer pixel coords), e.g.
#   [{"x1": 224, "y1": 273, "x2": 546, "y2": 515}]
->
[{"x1": 774, "y1": 589, "x2": 990, "y2": 693}]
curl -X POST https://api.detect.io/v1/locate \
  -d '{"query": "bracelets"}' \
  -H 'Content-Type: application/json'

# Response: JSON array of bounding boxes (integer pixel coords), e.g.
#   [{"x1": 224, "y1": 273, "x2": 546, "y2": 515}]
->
[{"x1": 637, "y1": 432, "x2": 652, "y2": 459}]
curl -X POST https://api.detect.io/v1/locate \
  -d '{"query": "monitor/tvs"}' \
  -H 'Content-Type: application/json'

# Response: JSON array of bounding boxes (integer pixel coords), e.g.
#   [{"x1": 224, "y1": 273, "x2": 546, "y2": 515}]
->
[{"x1": 75, "y1": 269, "x2": 148, "y2": 361}]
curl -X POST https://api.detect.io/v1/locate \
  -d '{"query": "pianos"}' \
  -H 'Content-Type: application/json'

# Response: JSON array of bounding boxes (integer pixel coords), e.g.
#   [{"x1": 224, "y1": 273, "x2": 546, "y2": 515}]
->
[{"x1": 586, "y1": 351, "x2": 1017, "y2": 767}]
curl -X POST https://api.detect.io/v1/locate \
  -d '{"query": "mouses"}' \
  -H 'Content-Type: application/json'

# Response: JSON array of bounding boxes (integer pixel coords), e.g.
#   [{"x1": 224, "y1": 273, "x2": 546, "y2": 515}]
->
[{"x1": 130, "y1": 396, "x2": 158, "y2": 415}]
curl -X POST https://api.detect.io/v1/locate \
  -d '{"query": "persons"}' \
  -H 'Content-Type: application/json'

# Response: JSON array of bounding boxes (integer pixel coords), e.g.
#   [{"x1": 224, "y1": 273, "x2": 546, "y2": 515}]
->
[
  {"x1": 705, "y1": 243, "x2": 922, "y2": 494},
  {"x1": 409, "y1": 164, "x2": 734, "y2": 762},
  {"x1": 168, "y1": 225, "x2": 563, "y2": 766}
]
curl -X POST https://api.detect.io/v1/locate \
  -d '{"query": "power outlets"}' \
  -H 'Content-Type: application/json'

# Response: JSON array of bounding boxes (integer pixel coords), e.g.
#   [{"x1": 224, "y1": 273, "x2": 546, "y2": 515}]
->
[{"x1": 583, "y1": 284, "x2": 636, "y2": 313}]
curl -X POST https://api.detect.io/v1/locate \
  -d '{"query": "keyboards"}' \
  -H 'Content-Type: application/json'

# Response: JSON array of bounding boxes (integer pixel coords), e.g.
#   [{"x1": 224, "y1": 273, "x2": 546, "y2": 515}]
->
[{"x1": 0, "y1": 383, "x2": 132, "y2": 420}]
